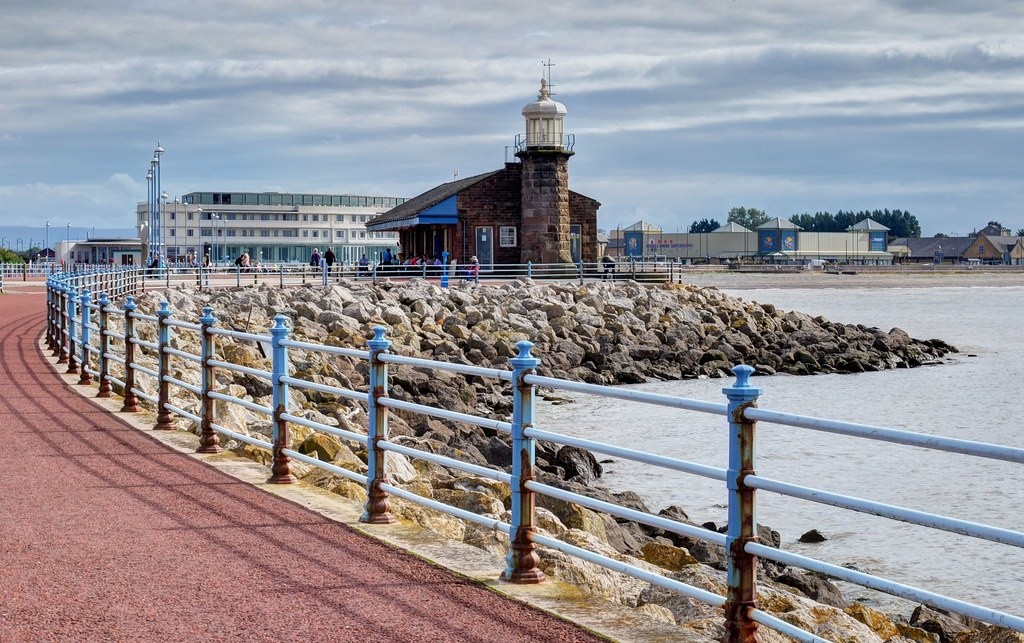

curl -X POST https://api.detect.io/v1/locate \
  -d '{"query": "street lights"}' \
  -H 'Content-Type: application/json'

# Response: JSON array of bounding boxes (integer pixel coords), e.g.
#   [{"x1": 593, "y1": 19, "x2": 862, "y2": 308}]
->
[
  {"x1": 950, "y1": 232, "x2": 960, "y2": 265},
  {"x1": 812, "y1": 223, "x2": 819, "y2": 258},
  {"x1": 196, "y1": 205, "x2": 203, "y2": 265},
  {"x1": 906, "y1": 229, "x2": 914, "y2": 265},
  {"x1": 46, "y1": 220, "x2": 50, "y2": 262},
  {"x1": 647, "y1": 224, "x2": 654, "y2": 255},
  {"x1": 183, "y1": 198, "x2": 189, "y2": 269},
  {"x1": 699, "y1": 227, "x2": 705, "y2": 264},
  {"x1": 633, "y1": 226, "x2": 639, "y2": 256},
  {"x1": 657, "y1": 225, "x2": 663, "y2": 260},
  {"x1": 223, "y1": 216, "x2": 228, "y2": 263},
  {"x1": 687, "y1": 225, "x2": 692, "y2": 258},
  {"x1": 211, "y1": 211, "x2": 219, "y2": 267},
  {"x1": 67, "y1": 222, "x2": 71, "y2": 263},
  {"x1": 161, "y1": 189, "x2": 167, "y2": 274},
  {"x1": 677, "y1": 224, "x2": 684, "y2": 261},
  {"x1": 2, "y1": 236, "x2": 22, "y2": 262},
  {"x1": 617, "y1": 223, "x2": 623, "y2": 256},
  {"x1": 174, "y1": 196, "x2": 179, "y2": 270},
  {"x1": 146, "y1": 141, "x2": 165, "y2": 279}
]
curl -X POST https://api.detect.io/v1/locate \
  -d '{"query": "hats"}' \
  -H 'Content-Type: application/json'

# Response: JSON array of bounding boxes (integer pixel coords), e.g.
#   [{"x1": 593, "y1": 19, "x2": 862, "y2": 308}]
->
[{"x1": 470, "y1": 256, "x2": 478, "y2": 260}]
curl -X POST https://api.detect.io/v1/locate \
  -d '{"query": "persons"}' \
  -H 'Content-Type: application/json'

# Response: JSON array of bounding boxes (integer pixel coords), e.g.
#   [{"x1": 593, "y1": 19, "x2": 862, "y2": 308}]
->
[
  {"x1": 390, "y1": 255, "x2": 399, "y2": 273},
  {"x1": 323, "y1": 246, "x2": 336, "y2": 276},
  {"x1": 203, "y1": 254, "x2": 210, "y2": 272},
  {"x1": 383, "y1": 248, "x2": 393, "y2": 276},
  {"x1": 358, "y1": 254, "x2": 372, "y2": 277},
  {"x1": 401, "y1": 255, "x2": 443, "y2": 276},
  {"x1": 310, "y1": 248, "x2": 320, "y2": 276},
  {"x1": 465, "y1": 256, "x2": 480, "y2": 283},
  {"x1": 601, "y1": 256, "x2": 616, "y2": 283},
  {"x1": 234, "y1": 253, "x2": 250, "y2": 272},
  {"x1": 191, "y1": 255, "x2": 199, "y2": 273}
]
